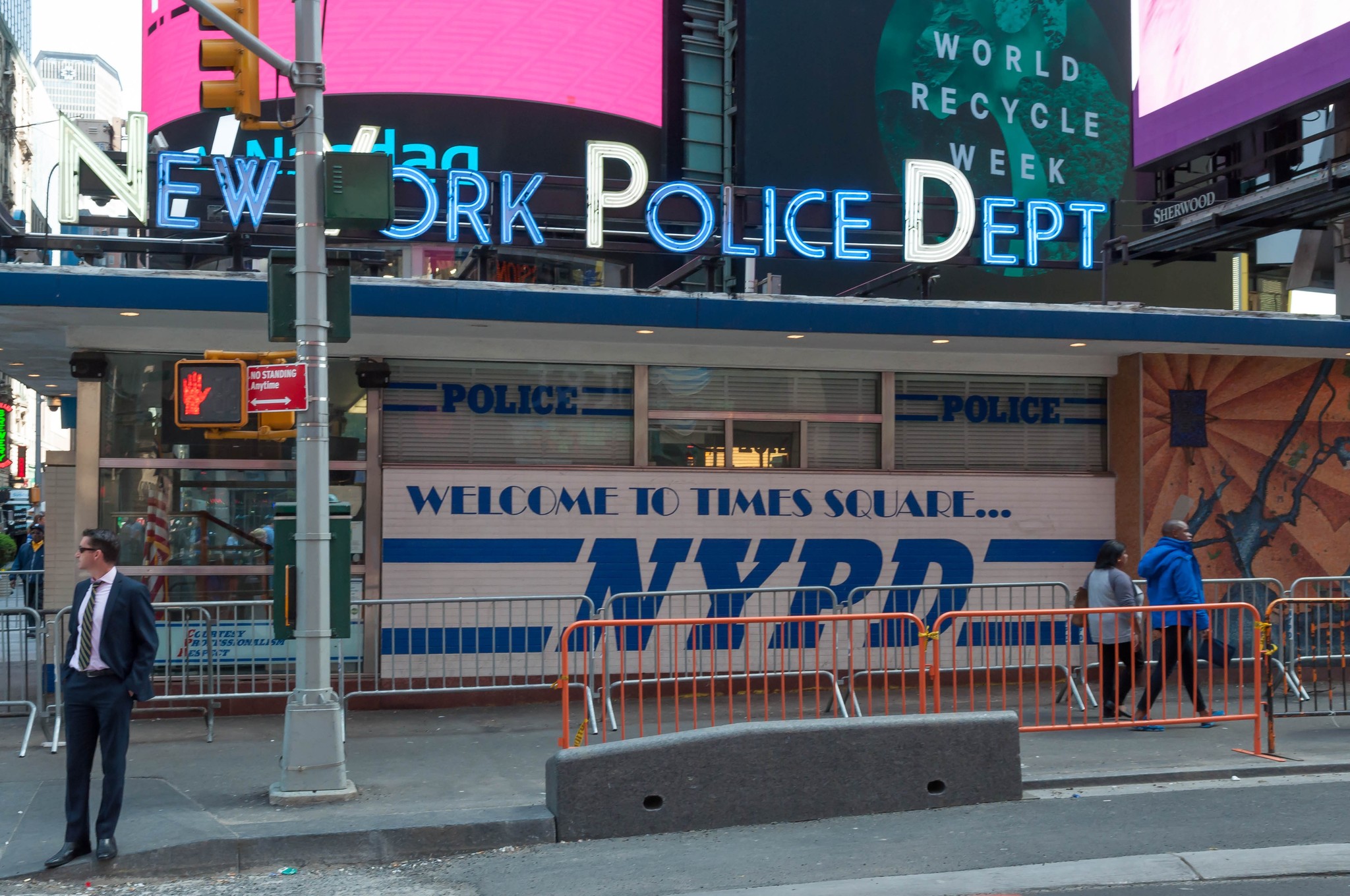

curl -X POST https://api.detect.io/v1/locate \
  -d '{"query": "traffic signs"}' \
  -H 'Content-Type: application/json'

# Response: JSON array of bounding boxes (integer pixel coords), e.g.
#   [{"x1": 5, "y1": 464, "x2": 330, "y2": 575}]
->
[{"x1": 246, "y1": 362, "x2": 309, "y2": 413}]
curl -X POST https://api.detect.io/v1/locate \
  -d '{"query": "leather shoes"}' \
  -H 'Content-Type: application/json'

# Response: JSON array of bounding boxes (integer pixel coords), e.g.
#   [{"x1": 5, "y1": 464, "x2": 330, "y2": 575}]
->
[
  {"x1": 95, "y1": 836, "x2": 116, "y2": 861},
  {"x1": 44, "y1": 838, "x2": 92, "y2": 868}
]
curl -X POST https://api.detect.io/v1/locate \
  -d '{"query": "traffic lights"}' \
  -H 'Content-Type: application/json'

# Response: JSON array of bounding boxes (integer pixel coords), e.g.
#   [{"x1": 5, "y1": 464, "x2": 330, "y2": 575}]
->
[
  {"x1": 198, "y1": 0, "x2": 261, "y2": 121},
  {"x1": 173, "y1": 357, "x2": 248, "y2": 430}
]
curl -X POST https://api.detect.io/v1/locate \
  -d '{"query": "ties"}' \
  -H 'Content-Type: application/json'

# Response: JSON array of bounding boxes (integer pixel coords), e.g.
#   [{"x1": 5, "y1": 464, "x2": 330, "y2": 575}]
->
[{"x1": 78, "y1": 581, "x2": 105, "y2": 670}]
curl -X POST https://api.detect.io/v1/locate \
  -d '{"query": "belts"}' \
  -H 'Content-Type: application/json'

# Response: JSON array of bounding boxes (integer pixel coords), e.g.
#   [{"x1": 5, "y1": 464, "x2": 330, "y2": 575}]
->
[{"x1": 85, "y1": 668, "x2": 114, "y2": 678}]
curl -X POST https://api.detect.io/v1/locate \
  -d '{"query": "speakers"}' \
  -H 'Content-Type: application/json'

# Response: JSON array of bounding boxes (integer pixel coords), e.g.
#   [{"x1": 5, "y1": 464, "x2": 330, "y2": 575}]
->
[
  {"x1": 71, "y1": 351, "x2": 106, "y2": 379},
  {"x1": 357, "y1": 362, "x2": 390, "y2": 387}
]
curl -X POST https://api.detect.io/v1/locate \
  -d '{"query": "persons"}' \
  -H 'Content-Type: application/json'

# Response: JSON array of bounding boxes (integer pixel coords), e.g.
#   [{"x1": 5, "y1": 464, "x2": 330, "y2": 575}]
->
[
  {"x1": 44, "y1": 527, "x2": 159, "y2": 869},
  {"x1": 1077, "y1": 541, "x2": 1144, "y2": 721},
  {"x1": 8, "y1": 523, "x2": 45, "y2": 637},
  {"x1": 1129, "y1": 520, "x2": 1226, "y2": 732},
  {"x1": 119, "y1": 513, "x2": 274, "y2": 621}
]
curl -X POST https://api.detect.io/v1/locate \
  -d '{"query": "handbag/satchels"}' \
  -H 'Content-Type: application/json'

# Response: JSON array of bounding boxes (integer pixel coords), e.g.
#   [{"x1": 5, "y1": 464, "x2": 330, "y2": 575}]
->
[{"x1": 1070, "y1": 571, "x2": 1096, "y2": 627}]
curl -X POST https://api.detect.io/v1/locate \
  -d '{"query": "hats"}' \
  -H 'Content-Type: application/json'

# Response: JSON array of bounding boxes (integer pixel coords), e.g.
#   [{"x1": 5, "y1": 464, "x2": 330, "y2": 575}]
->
[{"x1": 31, "y1": 523, "x2": 45, "y2": 534}]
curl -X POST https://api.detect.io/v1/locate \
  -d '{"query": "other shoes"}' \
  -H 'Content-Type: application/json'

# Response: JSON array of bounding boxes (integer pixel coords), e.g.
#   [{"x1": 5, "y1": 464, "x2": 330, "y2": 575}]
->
[
  {"x1": 1200, "y1": 710, "x2": 1225, "y2": 728},
  {"x1": 1131, "y1": 715, "x2": 1165, "y2": 732},
  {"x1": 1103, "y1": 709, "x2": 1133, "y2": 720},
  {"x1": 27, "y1": 632, "x2": 36, "y2": 639}
]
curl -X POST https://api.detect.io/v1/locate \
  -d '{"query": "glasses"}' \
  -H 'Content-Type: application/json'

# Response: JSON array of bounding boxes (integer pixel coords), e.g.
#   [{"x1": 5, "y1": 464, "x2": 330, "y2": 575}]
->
[
  {"x1": 79, "y1": 547, "x2": 99, "y2": 553},
  {"x1": 31, "y1": 532, "x2": 43, "y2": 535}
]
